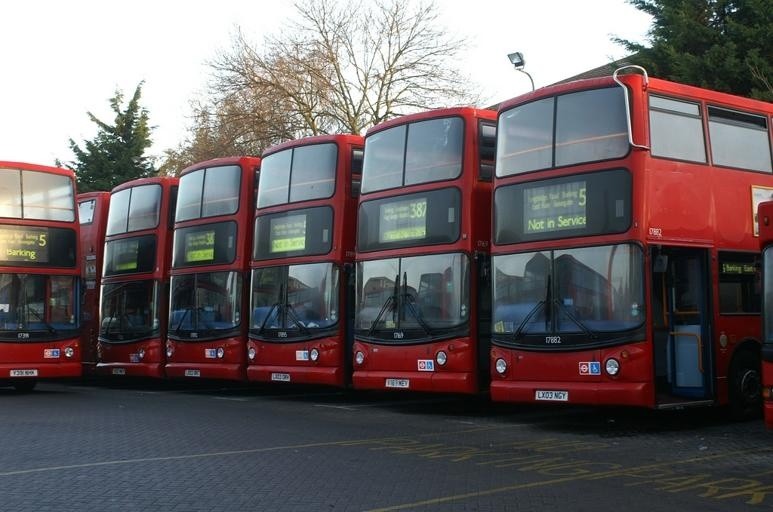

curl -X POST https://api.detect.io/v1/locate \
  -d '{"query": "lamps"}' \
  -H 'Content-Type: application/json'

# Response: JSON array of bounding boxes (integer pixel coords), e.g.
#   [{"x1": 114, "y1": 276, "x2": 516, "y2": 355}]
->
[{"x1": 506, "y1": 51, "x2": 534, "y2": 89}]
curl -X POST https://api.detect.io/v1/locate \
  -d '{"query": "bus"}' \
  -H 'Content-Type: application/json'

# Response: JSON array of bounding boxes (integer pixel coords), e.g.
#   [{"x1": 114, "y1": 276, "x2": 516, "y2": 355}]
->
[
  {"x1": 489, "y1": 64, "x2": 772, "y2": 422},
  {"x1": 0, "y1": 161, "x2": 83, "y2": 393},
  {"x1": 94, "y1": 177, "x2": 179, "y2": 379},
  {"x1": 165, "y1": 157, "x2": 262, "y2": 381},
  {"x1": 74, "y1": 192, "x2": 111, "y2": 378},
  {"x1": 756, "y1": 188, "x2": 773, "y2": 442},
  {"x1": 26, "y1": 249, "x2": 617, "y2": 325},
  {"x1": 246, "y1": 134, "x2": 365, "y2": 386},
  {"x1": 350, "y1": 108, "x2": 500, "y2": 396}
]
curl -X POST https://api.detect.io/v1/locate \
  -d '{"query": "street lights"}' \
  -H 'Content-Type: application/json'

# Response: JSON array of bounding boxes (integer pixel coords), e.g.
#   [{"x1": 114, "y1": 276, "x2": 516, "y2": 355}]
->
[{"x1": 507, "y1": 50, "x2": 540, "y2": 92}]
